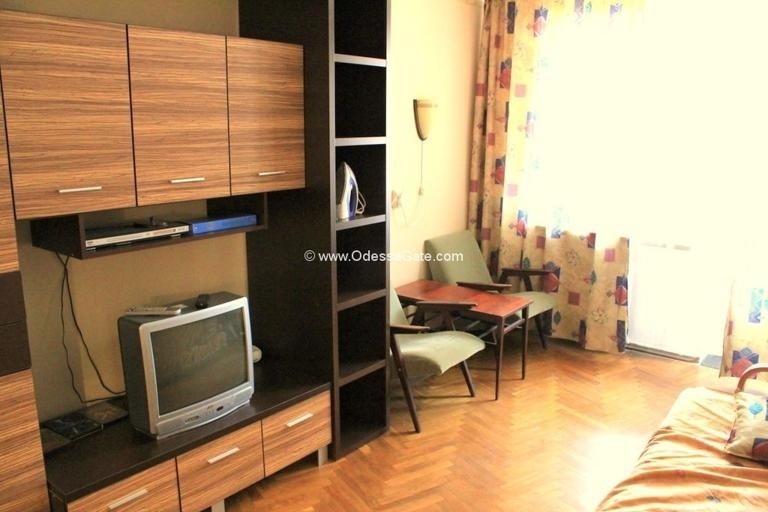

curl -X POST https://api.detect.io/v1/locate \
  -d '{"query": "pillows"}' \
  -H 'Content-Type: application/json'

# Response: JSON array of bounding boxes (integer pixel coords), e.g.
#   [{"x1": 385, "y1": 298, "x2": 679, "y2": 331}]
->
[{"x1": 724, "y1": 390, "x2": 768, "y2": 463}]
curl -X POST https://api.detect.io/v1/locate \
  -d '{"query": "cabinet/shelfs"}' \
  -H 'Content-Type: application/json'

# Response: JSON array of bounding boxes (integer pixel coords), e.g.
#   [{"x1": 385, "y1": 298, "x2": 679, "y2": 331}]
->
[
  {"x1": 227, "y1": 34, "x2": 306, "y2": 204},
  {"x1": 1, "y1": 10, "x2": 137, "y2": 235},
  {"x1": 208, "y1": 1, "x2": 389, "y2": 462},
  {"x1": 66, "y1": 386, "x2": 334, "y2": 511},
  {"x1": 126, "y1": 21, "x2": 231, "y2": 213}
]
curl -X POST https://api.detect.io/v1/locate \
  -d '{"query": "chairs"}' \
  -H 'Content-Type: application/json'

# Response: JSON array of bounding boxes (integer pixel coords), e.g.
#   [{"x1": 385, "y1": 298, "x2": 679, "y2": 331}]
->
[{"x1": 390, "y1": 227, "x2": 559, "y2": 434}]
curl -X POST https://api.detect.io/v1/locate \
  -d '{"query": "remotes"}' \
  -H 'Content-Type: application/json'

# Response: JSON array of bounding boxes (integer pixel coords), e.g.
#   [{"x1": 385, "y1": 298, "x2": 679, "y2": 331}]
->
[
  {"x1": 124, "y1": 305, "x2": 181, "y2": 316},
  {"x1": 195, "y1": 292, "x2": 210, "y2": 309}
]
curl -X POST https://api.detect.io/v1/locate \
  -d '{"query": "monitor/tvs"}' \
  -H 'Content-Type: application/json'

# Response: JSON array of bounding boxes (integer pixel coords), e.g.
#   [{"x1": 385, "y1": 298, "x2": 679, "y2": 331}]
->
[{"x1": 118, "y1": 291, "x2": 255, "y2": 441}]
